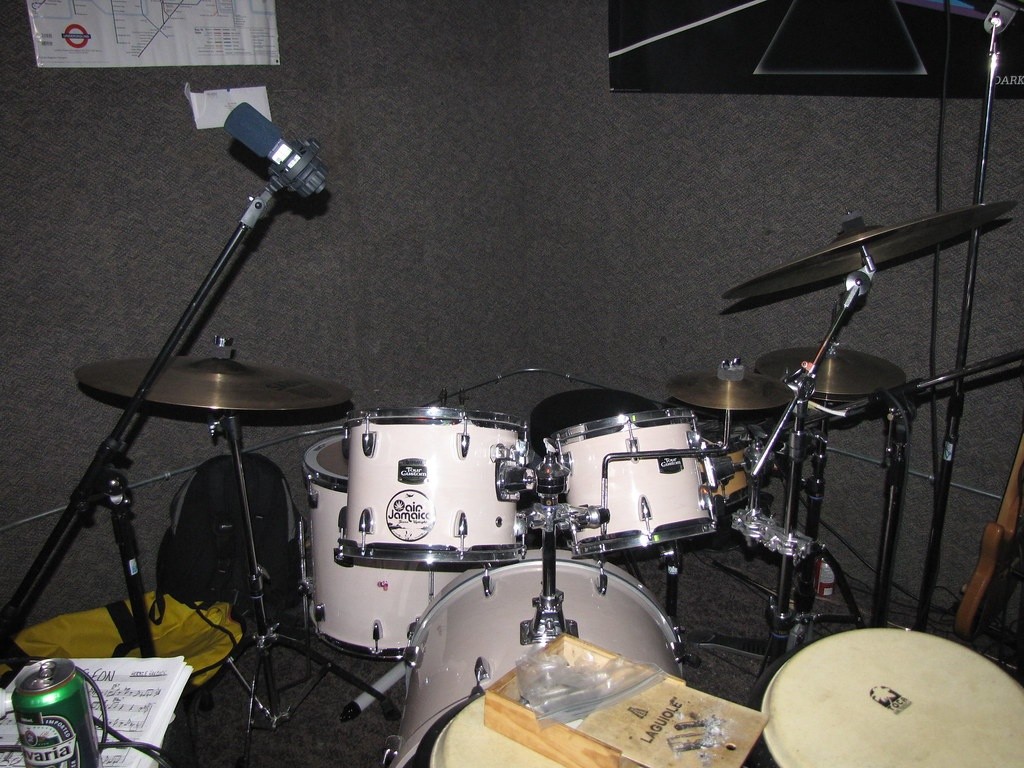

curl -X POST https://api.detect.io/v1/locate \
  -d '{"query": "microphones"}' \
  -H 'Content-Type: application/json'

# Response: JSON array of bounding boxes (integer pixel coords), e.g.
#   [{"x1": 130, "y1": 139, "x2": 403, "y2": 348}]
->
[{"x1": 223, "y1": 101, "x2": 330, "y2": 199}]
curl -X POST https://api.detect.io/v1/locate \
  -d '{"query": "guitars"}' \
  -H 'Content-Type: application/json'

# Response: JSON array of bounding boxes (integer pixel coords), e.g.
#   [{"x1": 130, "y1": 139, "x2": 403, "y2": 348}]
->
[{"x1": 953, "y1": 434, "x2": 1024, "y2": 636}]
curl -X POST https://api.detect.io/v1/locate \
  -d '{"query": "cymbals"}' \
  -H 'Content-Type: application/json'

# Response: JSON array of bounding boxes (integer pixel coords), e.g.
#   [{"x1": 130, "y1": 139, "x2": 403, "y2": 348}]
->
[
  {"x1": 720, "y1": 195, "x2": 1020, "y2": 305},
  {"x1": 72, "y1": 358, "x2": 354, "y2": 416},
  {"x1": 756, "y1": 347, "x2": 903, "y2": 405},
  {"x1": 661, "y1": 368, "x2": 793, "y2": 412}
]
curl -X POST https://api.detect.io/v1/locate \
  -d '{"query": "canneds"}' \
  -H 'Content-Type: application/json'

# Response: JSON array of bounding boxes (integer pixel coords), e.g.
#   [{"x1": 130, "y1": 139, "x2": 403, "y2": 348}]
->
[
  {"x1": 11, "y1": 658, "x2": 104, "y2": 768},
  {"x1": 814, "y1": 556, "x2": 834, "y2": 595}
]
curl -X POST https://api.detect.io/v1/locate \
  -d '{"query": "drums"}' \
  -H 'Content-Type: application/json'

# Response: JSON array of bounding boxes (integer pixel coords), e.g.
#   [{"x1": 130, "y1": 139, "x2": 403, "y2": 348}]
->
[
  {"x1": 342, "y1": 409, "x2": 535, "y2": 565},
  {"x1": 384, "y1": 548, "x2": 684, "y2": 768},
  {"x1": 742, "y1": 627, "x2": 1024, "y2": 767},
  {"x1": 303, "y1": 431, "x2": 468, "y2": 661},
  {"x1": 550, "y1": 405, "x2": 719, "y2": 558}
]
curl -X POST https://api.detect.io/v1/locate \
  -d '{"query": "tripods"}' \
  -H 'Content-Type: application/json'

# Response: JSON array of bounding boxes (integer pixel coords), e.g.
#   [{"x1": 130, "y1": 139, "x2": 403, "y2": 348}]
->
[
  {"x1": 703, "y1": 252, "x2": 876, "y2": 710},
  {"x1": 196, "y1": 417, "x2": 403, "y2": 768}
]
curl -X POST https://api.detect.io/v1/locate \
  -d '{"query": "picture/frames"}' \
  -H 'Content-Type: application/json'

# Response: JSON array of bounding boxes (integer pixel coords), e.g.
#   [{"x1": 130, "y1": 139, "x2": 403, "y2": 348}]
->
[{"x1": 607, "y1": 0, "x2": 1024, "y2": 100}]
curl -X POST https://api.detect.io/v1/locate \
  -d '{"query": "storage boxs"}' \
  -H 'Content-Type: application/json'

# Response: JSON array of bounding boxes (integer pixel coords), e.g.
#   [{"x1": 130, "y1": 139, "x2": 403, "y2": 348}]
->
[{"x1": 484, "y1": 631, "x2": 770, "y2": 768}]
